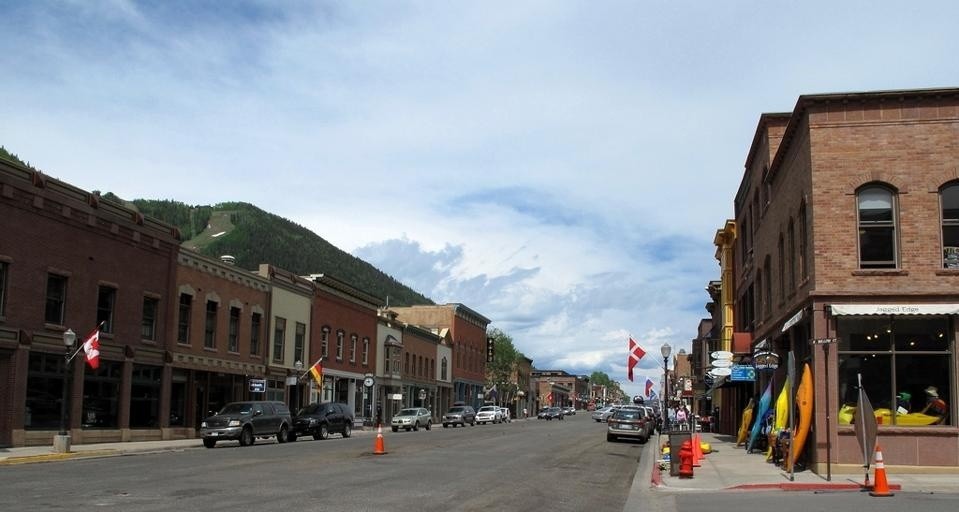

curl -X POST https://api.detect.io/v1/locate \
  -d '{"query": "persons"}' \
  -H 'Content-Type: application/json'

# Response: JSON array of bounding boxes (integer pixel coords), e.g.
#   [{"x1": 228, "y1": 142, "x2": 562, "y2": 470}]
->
[
  {"x1": 667, "y1": 404, "x2": 689, "y2": 424},
  {"x1": 523, "y1": 407, "x2": 528, "y2": 418}
]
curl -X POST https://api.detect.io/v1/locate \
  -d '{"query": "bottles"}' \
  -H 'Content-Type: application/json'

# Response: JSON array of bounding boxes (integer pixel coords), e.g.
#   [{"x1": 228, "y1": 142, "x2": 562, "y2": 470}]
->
[{"x1": 924, "y1": 386, "x2": 938, "y2": 397}]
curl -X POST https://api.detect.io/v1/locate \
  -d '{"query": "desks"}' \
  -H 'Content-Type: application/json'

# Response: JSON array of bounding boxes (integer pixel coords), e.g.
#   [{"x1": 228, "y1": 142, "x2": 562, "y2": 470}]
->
[
  {"x1": 56, "y1": 326, "x2": 79, "y2": 435},
  {"x1": 483, "y1": 386, "x2": 487, "y2": 406},
  {"x1": 659, "y1": 342, "x2": 673, "y2": 432},
  {"x1": 293, "y1": 359, "x2": 303, "y2": 413}
]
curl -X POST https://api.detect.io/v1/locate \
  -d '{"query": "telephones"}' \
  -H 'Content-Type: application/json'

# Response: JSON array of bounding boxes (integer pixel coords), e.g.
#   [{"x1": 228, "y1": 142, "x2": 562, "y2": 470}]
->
[
  {"x1": 710, "y1": 351, "x2": 735, "y2": 359},
  {"x1": 731, "y1": 366, "x2": 757, "y2": 382},
  {"x1": 710, "y1": 359, "x2": 735, "y2": 367},
  {"x1": 710, "y1": 367, "x2": 733, "y2": 376}
]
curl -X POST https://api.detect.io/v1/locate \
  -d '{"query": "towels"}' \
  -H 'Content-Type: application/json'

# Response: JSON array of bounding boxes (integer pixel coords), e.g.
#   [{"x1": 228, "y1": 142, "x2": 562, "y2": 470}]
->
[{"x1": 669, "y1": 431, "x2": 692, "y2": 476}]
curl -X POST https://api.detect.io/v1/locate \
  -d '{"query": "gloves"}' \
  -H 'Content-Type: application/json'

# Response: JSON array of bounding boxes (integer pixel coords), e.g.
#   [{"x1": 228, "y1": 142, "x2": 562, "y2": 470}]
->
[
  {"x1": 838, "y1": 404, "x2": 942, "y2": 425},
  {"x1": 766, "y1": 378, "x2": 790, "y2": 461},
  {"x1": 745, "y1": 379, "x2": 772, "y2": 454},
  {"x1": 734, "y1": 398, "x2": 754, "y2": 446},
  {"x1": 787, "y1": 362, "x2": 814, "y2": 472}
]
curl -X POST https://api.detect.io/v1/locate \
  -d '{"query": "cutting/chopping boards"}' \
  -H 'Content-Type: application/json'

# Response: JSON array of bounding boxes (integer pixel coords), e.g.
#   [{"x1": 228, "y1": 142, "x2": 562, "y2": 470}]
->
[{"x1": 677, "y1": 440, "x2": 694, "y2": 478}]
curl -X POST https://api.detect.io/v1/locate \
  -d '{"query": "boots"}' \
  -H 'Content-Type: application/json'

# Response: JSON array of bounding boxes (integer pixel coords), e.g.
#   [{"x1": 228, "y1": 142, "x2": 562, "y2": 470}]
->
[
  {"x1": 390, "y1": 407, "x2": 433, "y2": 432},
  {"x1": 441, "y1": 405, "x2": 476, "y2": 428},
  {"x1": 293, "y1": 402, "x2": 356, "y2": 441},
  {"x1": 197, "y1": 398, "x2": 292, "y2": 449},
  {"x1": 475, "y1": 405, "x2": 502, "y2": 425}
]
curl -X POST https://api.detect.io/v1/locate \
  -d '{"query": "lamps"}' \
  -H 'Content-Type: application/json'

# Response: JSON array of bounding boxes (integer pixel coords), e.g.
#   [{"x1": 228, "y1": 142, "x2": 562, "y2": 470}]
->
[
  {"x1": 666, "y1": 415, "x2": 716, "y2": 435},
  {"x1": 744, "y1": 430, "x2": 806, "y2": 471}
]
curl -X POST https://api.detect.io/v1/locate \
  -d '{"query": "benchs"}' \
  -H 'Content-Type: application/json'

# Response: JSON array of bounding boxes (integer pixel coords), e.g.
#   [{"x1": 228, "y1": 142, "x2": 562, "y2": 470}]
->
[
  {"x1": 546, "y1": 393, "x2": 553, "y2": 402},
  {"x1": 649, "y1": 389, "x2": 656, "y2": 401},
  {"x1": 627, "y1": 337, "x2": 646, "y2": 381},
  {"x1": 645, "y1": 377, "x2": 654, "y2": 397},
  {"x1": 488, "y1": 384, "x2": 497, "y2": 398},
  {"x1": 82, "y1": 325, "x2": 101, "y2": 369},
  {"x1": 310, "y1": 357, "x2": 323, "y2": 390}
]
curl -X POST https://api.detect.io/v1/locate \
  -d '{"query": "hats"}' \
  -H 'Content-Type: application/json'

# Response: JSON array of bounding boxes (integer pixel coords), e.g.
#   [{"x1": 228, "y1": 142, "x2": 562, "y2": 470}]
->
[
  {"x1": 546, "y1": 407, "x2": 564, "y2": 420},
  {"x1": 587, "y1": 403, "x2": 615, "y2": 412},
  {"x1": 537, "y1": 406, "x2": 576, "y2": 419},
  {"x1": 500, "y1": 407, "x2": 511, "y2": 424},
  {"x1": 607, "y1": 404, "x2": 657, "y2": 444},
  {"x1": 591, "y1": 406, "x2": 615, "y2": 423}
]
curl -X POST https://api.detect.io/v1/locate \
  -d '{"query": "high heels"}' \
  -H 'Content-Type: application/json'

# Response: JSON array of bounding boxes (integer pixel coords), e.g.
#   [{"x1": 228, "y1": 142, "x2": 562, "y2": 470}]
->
[
  {"x1": 868, "y1": 445, "x2": 896, "y2": 497},
  {"x1": 371, "y1": 422, "x2": 388, "y2": 454},
  {"x1": 687, "y1": 438, "x2": 701, "y2": 467},
  {"x1": 696, "y1": 434, "x2": 705, "y2": 459}
]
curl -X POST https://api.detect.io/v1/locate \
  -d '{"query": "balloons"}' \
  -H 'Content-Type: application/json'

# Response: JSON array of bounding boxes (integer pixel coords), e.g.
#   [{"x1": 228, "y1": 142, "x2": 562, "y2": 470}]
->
[{"x1": 363, "y1": 377, "x2": 375, "y2": 387}]
